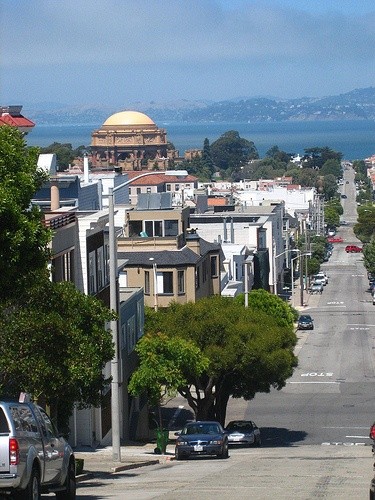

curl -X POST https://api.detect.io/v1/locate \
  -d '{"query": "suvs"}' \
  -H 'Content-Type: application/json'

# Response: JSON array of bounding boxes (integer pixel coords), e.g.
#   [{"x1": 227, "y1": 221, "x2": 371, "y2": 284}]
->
[
  {"x1": 344, "y1": 163, "x2": 353, "y2": 167},
  {"x1": 0, "y1": 401, "x2": 76, "y2": 500}
]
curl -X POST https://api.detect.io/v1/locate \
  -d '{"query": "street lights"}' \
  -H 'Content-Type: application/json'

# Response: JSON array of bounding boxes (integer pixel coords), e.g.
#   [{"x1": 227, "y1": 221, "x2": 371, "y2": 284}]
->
[
  {"x1": 273, "y1": 249, "x2": 300, "y2": 295},
  {"x1": 291, "y1": 253, "x2": 312, "y2": 307},
  {"x1": 108, "y1": 169, "x2": 189, "y2": 462}
]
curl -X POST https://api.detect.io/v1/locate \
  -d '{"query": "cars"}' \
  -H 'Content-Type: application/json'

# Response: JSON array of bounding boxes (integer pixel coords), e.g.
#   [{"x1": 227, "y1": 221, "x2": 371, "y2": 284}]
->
[
  {"x1": 345, "y1": 245, "x2": 362, "y2": 253},
  {"x1": 324, "y1": 275, "x2": 329, "y2": 284},
  {"x1": 225, "y1": 419, "x2": 261, "y2": 448},
  {"x1": 369, "y1": 421, "x2": 375, "y2": 500},
  {"x1": 346, "y1": 181, "x2": 349, "y2": 184},
  {"x1": 329, "y1": 230, "x2": 335, "y2": 236},
  {"x1": 319, "y1": 272, "x2": 323, "y2": 274},
  {"x1": 298, "y1": 315, "x2": 314, "y2": 330},
  {"x1": 175, "y1": 421, "x2": 228, "y2": 458},
  {"x1": 340, "y1": 220, "x2": 348, "y2": 225},
  {"x1": 327, "y1": 237, "x2": 343, "y2": 242},
  {"x1": 312, "y1": 282, "x2": 323, "y2": 291},
  {"x1": 316, "y1": 274, "x2": 326, "y2": 286},
  {"x1": 341, "y1": 195, "x2": 347, "y2": 198}
]
